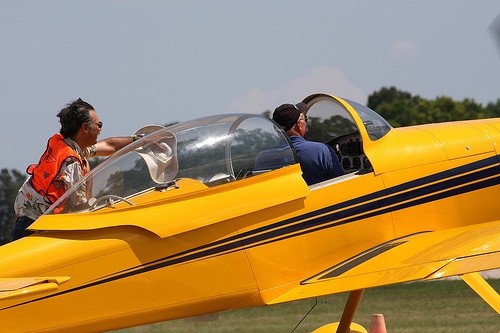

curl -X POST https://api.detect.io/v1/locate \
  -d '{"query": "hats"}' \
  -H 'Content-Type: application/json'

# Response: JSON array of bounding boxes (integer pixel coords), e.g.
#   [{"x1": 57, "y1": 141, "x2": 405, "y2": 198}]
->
[
  {"x1": 273, "y1": 102, "x2": 307, "y2": 132},
  {"x1": 130, "y1": 125, "x2": 179, "y2": 184}
]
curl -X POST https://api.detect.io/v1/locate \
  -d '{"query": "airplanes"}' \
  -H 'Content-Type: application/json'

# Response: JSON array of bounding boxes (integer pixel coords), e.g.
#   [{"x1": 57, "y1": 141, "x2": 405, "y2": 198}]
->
[{"x1": 0, "y1": 93, "x2": 499, "y2": 333}]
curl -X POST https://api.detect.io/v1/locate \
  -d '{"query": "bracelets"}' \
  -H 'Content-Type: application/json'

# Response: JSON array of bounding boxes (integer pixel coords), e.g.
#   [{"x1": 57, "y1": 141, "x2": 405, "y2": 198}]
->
[{"x1": 133, "y1": 134, "x2": 145, "y2": 142}]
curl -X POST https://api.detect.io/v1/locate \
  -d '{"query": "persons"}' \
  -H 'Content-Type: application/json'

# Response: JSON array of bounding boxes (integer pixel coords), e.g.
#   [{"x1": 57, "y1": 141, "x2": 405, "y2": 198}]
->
[
  {"x1": 256, "y1": 103, "x2": 345, "y2": 186},
  {"x1": 9, "y1": 98, "x2": 164, "y2": 242}
]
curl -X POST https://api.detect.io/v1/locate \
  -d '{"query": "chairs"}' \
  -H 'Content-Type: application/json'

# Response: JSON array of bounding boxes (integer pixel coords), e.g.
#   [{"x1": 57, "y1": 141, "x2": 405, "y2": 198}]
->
[{"x1": 254, "y1": 146, "x2": 295, "y2": 172}]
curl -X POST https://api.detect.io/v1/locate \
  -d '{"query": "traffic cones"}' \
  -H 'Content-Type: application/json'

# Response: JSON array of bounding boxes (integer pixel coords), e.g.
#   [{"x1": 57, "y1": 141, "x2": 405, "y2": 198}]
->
[{"x1": 369, "y1": 313, "x2": 386, "y2": 333}]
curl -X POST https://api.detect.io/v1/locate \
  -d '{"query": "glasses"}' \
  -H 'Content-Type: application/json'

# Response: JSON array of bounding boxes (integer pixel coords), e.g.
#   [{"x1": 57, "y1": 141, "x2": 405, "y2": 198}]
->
[
  {"x1": 90, "y1": 121, "x2": 105, "y2": 128},
  {"x1": 298, "y1": 116, "x2": 308, "y2": 124}
]
